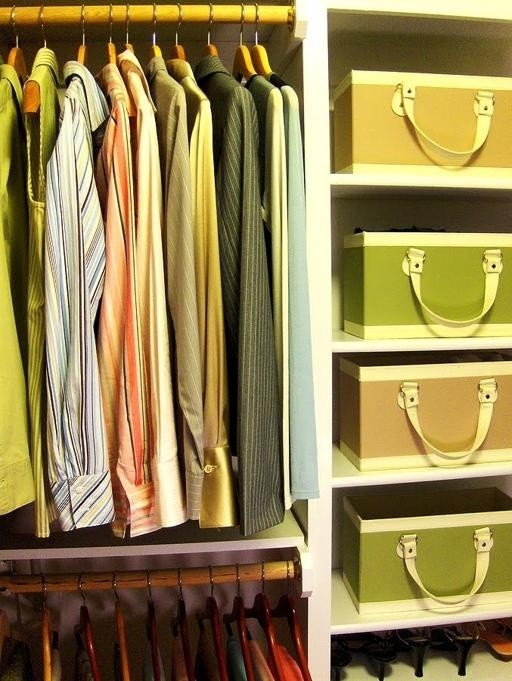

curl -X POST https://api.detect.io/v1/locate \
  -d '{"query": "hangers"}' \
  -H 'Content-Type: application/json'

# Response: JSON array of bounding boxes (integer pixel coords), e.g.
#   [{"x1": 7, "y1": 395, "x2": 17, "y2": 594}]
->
[{"x1": 7, "y1": 3, "x2": 273, "y2": 113}]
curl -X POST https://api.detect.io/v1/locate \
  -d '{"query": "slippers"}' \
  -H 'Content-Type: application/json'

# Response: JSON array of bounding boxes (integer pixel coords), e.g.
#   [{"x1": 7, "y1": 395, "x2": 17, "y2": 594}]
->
[{"x1": 462, "y1": 616, "x2": 512, "y2": 662}]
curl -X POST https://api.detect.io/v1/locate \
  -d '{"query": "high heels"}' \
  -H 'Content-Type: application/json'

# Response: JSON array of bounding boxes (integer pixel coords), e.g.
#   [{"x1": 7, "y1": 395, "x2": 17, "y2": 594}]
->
[{"x1": 331, "y1": 620, "x2": 479, "y2": 681}]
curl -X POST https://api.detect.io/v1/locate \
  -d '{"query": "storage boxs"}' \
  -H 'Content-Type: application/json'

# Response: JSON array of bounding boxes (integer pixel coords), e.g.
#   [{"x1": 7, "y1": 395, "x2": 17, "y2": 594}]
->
[
  {"x1": 342, "y1": 486, "x2": 512, "y2": 615},
  {"x1": 333, "y1": 69, "x2": 512, "y2": 179},
  {"x1": 343, "y1": 231, "x2": 512, "y2": 340},
  {"x1": 339, "y1": 356, "x2": 512, "y2": 472}
]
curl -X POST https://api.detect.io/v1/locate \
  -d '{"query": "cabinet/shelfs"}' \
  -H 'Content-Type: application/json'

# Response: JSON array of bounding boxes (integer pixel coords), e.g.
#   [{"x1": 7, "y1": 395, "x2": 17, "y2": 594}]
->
[
  {"x1": 0, "y1": 0, "x2": 309, "y2": 681},
  {"x1": 328, "y1": 0, "x2": 512, "y2": 681}
]
